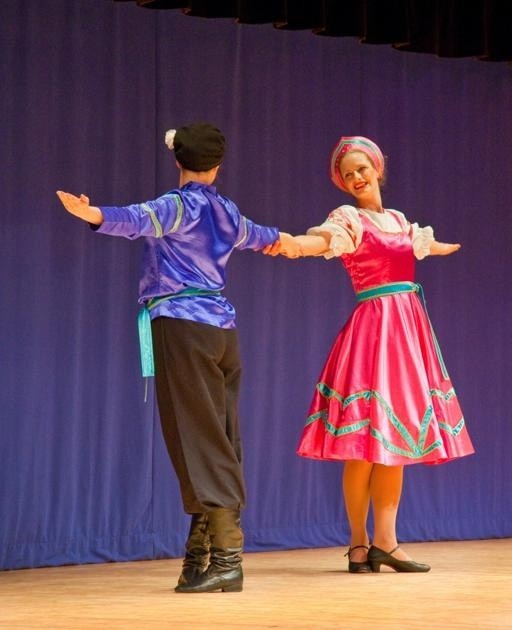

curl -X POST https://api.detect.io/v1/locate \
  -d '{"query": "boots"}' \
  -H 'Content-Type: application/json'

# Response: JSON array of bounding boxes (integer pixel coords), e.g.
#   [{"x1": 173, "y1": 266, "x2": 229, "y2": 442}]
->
[{"x1": 175, "y1": 508, "x2": 243, "y2": 593}]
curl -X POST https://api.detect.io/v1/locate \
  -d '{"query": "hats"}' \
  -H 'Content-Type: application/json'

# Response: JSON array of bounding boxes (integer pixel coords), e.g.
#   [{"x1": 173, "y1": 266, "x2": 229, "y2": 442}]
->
[
  {"x1": 328, "y1": 136, "x2": 384, "y2": 193},
  {"x1": 165, "y1": 121, "x2": 227, "y2": 171}
]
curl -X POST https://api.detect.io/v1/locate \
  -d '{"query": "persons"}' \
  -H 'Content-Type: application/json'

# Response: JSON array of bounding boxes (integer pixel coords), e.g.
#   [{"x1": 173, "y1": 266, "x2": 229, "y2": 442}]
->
[
  {"x1": 261, "y1": 137, "x2": 463, "y2": 573},
  {"x1": 55, "y1": 123, "x2": 304, "y2": 593}
]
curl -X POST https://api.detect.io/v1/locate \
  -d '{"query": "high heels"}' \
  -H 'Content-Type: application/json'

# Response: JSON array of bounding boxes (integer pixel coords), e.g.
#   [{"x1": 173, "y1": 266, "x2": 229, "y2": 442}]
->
[
  {"x1": 344, "y1": 545, "x2": 372, "y2": 573},
  {"x1": 367, "y1": 545, "x2": 431, "y2": 572}
]
